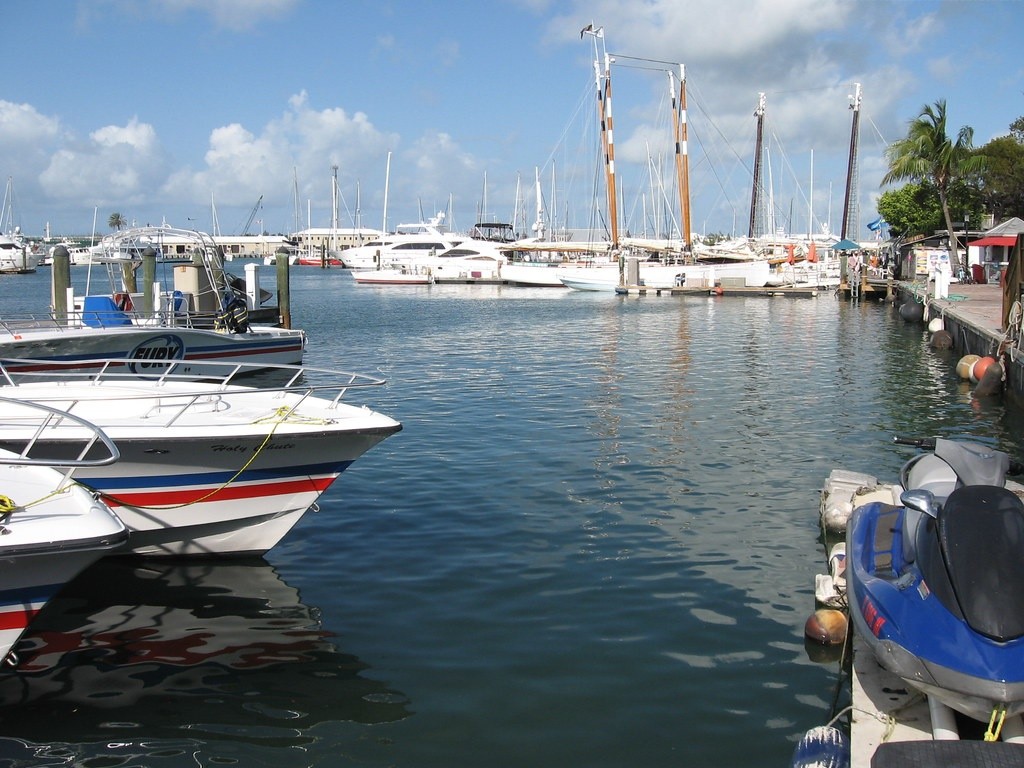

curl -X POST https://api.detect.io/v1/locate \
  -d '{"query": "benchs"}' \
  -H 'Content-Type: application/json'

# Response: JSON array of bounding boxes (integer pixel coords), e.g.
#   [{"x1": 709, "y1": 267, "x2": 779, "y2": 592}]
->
[{"x1": 82, "y1": 297, "x2": 133, "y2": 327}]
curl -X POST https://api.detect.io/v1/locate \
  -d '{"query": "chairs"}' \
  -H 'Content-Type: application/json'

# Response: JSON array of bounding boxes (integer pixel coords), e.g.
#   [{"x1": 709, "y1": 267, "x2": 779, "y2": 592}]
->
[
  {"x1": 903, "y1": 456, "x2": 957, "y2": 562},
  {"x1": 172, "y1": 290, "x2": 183, "y2": 311}
]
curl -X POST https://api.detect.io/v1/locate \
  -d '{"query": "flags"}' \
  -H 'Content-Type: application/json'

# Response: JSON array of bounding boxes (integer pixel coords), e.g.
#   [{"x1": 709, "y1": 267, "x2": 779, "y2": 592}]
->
[
  {"x1": 867, "y1": 217, "x2": 881, "y2": 231},
  {"x1": 256, "y1": 219, "x2": 261, "y2": 224},
  {"x1": 580, "y1": 25, "x2": 592, "y2": 39}
]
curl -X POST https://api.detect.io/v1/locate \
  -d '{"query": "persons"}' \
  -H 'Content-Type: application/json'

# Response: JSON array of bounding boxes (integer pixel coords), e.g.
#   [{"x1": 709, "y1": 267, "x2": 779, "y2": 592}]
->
[{"x1": 848, "y1": 252, "x2": 889, "y2": 279}]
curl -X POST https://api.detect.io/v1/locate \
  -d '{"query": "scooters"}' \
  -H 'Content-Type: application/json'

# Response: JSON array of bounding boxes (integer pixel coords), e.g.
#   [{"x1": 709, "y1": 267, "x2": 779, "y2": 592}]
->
[{"x1": 845, "y1": 429, "x2": 1024, "y2": 727}]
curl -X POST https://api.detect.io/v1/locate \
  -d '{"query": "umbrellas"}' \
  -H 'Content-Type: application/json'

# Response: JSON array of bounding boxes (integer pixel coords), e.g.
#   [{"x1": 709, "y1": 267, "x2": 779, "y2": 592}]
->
[
  {"x1": 832, "y1": 239, "x2": 863, "y2": 250},
  {"x1": 788, "y1": 244, "x2": 795, "y2": 281},
  {"x1": 807, "y1": 241, "x2": 818, "y2": 263}
]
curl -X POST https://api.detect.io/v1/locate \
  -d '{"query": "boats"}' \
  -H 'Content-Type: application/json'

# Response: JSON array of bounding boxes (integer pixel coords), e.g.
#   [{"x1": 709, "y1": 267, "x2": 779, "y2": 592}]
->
[
  {"x1": 0, "y1": 394, "x2": 131, "y2": 670},
  {"x1": 0, "y1": 357, "x2": 405, "y2": 561},
  {"x1": 1, "y1": 222, "x2": 309, "y2": 385}
]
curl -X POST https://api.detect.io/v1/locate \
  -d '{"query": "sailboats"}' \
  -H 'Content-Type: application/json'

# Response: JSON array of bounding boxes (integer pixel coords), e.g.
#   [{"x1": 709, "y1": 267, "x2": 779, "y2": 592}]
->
[{"x1": 0, "y1": 24, "x2": 870, "y2": 300}]
